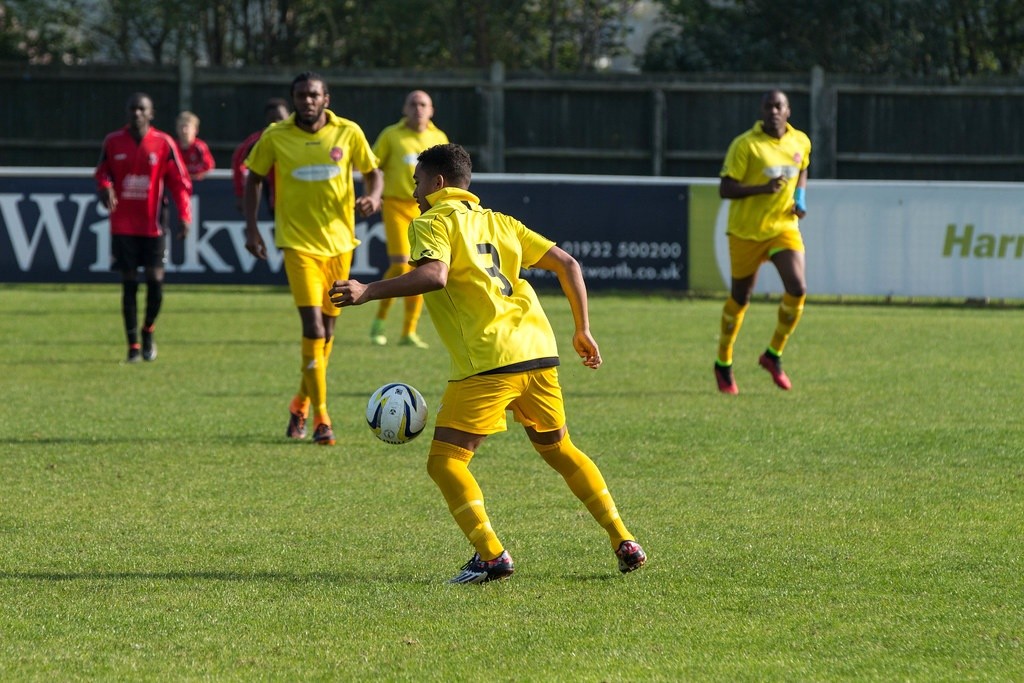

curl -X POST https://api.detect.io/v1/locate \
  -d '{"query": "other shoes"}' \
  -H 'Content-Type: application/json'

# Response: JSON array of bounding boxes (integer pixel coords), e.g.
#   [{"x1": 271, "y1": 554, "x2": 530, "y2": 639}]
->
[
  {"x1": 399, "y1": 335, "x2": 430, "y2": 349},
  {"x1": 127, "y1": 347, "x2": 140, "y2": 363},
  {"x1": 141, "y1": 330, "x2": 157, "y2": 360},
  {"x1": 370, "y1": 319, "x2": 386, "y2": 343}
]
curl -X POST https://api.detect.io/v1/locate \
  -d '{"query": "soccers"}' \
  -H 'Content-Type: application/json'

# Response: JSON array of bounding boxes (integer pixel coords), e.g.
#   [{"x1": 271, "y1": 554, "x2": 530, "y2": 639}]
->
[{"x1": 364, "y1": 383, "x2": 427, "y2": 445}]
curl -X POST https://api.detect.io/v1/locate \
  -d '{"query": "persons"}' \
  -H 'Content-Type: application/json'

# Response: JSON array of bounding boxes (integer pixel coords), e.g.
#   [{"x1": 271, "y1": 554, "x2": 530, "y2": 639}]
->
[
  {"x1": 327, "y1": 143, "x2": 645, "y2": 583},
  {"x1": 362, "y1": 89, "x2": 447, "y2": 349},
  {"x1": 244, "y1": 69, "x2": 385, "y2": 445},
  {"x1": 713, "y1": 88, "x2": 813, "y2": 396},
  {"x1": 234, "y1": 98, "x2": 292, "y2": 214},
  {"x1": 92, "y1": 92, "x2": 190, "y2": 361},
  {"x1": 171, "y1": 110, "x2": 215, "y2": 175}
]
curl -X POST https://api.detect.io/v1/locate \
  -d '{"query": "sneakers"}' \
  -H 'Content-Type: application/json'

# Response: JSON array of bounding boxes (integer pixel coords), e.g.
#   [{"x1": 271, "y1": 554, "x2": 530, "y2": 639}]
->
[
  {"x1": 443, "y1": 549, "x2": 515, "y2": 585},
  {"x1": 759, "y1": 348, "x2": 792, "y2": 391},
  {"x1": 312, "y1": 423, "x2": 335, "y2": 445},
  {"x1": 715, "y1": 361, "x2": 739, "y2": 395},
  {"x1": 287, "y1": 413, "x2": 308, "y2": 439},
  {"x1": 615, "y1": 540, "x2": 647, "y2": 574}
]
